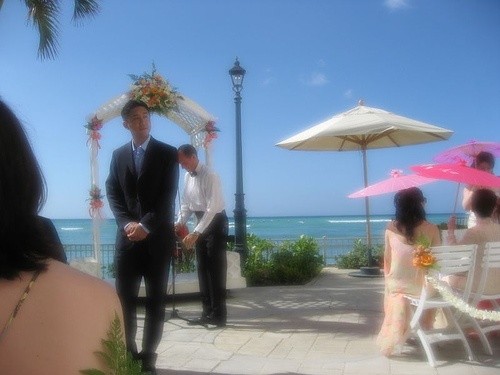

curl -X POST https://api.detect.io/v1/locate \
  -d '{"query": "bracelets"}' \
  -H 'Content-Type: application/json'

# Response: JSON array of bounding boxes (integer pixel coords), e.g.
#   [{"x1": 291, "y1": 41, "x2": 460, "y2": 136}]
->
[{"x1": 192, "y1": 229, "x2": 200, "y2": 236}]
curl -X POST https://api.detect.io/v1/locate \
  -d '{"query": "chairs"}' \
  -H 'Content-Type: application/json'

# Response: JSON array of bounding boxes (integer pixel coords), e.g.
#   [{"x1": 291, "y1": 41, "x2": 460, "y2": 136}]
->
[{"x1": 405, "y1": 242, "x2": 500, "y2": 370}]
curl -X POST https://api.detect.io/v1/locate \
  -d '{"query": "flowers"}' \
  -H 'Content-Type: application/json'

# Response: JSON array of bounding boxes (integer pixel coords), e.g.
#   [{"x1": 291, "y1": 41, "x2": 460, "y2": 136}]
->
[
  {"x1": 84, "y1": 115, "x2": 104, "y2": 148},
  {"x1": 413, "y1": 232, "x2": 438, "y2": 271},
  {"x1": 87, "y1": 184, "x2": 106, "y2": 217},
  {"x1": 202, "y1": 121, "x2": 220, "y2": 146},
  {"x1": 128, "y1": 59, "x2": 185, "y2": 118}
]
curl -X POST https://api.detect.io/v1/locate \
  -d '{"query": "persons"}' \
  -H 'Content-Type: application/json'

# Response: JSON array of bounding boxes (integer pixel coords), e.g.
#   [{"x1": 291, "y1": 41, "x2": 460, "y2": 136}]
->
[
  {"x1": 460, "y1": 151, "x2": 500, "y2": 229},
  {"x1": 104, "y1": 99, "x2": 179, "y2": 375},
  {"x1": 0, "y1": 100, "x2": 129, "y2": 375},
  {"x1": 374, "y1": 186, "x2": 456, "y2": 357},
  {"x1": 174, "y1": 144, "x2": 229, "y2": 329},
  {"x1": 431, "y1": 188, "x2": 500, "y2": 346}
]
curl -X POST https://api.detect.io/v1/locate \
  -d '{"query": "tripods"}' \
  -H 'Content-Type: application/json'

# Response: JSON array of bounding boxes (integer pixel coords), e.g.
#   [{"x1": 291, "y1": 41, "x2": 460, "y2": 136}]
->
[{"x1": 164, "y1": 252, "x2": 208, "y2": 328}]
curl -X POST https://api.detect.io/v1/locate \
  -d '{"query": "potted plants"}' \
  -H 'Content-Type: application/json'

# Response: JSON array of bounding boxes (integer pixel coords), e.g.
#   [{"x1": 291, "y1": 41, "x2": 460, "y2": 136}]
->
[{"x1": 270, "y1": 237, "x2": 323, "y2": 285}]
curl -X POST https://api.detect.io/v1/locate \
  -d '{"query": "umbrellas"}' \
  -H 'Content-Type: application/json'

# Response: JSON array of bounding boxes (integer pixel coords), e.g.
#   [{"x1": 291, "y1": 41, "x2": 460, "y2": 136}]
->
[
  {"x1": 411, "y1": 162, "x2": 500, "y2": 217},
  {"x1": 348, "y1": 168, "x2": 439, "y2": 198},
  {"x1": 273, "y1": 99, "x2": 457, "y2": 267},
  {"x1": 431, "y1": 139, "x2": 499, "y2": 169}
]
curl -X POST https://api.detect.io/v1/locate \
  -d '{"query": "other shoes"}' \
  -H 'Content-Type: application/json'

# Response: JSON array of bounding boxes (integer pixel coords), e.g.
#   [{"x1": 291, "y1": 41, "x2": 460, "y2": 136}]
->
[
  {"x1": 140, "y1": 367, "x2": 156, "y2": 375},
  {"x1": 202, "y1": 313, "x2": 225, "y2": 326},
  {"x1": 407, "y1": 335, "x2": 418, "y2": 345},
  {"x1": 190, "y1": 316, "x2": 205, "y2": 325}
]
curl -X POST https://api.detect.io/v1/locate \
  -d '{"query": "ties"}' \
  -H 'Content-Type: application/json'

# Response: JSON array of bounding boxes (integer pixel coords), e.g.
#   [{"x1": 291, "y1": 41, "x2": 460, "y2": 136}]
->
[{"x1": 134, "y1": 148, "x2": 142, "y2": 175}]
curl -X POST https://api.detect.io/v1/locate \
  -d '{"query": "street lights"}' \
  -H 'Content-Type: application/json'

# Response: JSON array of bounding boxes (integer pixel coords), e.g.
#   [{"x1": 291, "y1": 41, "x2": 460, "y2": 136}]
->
[{"x1": 227, "y1": 56, "x2": 249, "y2": 263}]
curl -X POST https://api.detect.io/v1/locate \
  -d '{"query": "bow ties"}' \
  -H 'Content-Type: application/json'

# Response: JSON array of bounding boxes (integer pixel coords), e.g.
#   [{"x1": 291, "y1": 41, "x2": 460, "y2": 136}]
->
[{"x1": 190, "y1": 171, "x2": 196, "y2": 177}]
